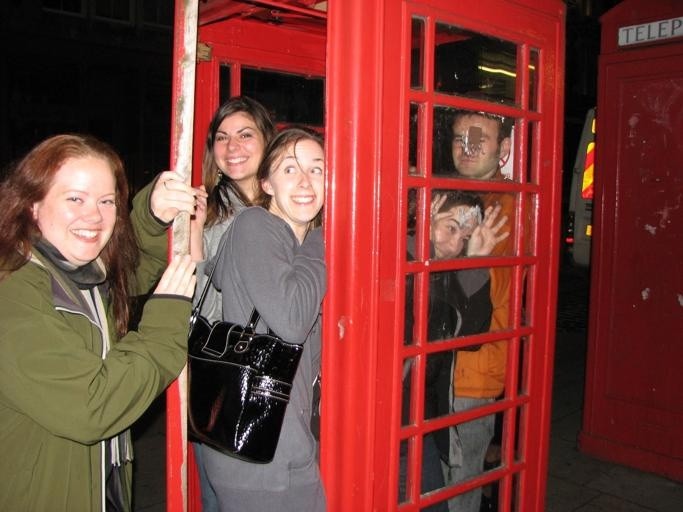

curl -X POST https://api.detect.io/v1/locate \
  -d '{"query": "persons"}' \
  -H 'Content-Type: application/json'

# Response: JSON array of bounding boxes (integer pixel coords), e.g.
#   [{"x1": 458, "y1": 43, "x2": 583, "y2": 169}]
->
[
  {"x1": 190, "y1": 97, "x2": 277, "y2": 266},
  {"x1": 0, "y1": 134, "x2": 197, "y2": 512},
  {"x1": 439, "y1": 91, "x2": 531, "y2": 512},
  {"x1": 398, "y1": 167, "x2": 511, "y2": 512},
  {"x1": 190, "y1": 126, "x2": 328, "y2": 512}
]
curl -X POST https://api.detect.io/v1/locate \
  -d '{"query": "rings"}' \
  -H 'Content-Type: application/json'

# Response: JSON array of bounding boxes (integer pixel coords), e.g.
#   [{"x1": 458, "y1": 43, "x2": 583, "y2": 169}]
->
[{"x1": 163, "y1": 182, "x2": 167, "y2": 190}]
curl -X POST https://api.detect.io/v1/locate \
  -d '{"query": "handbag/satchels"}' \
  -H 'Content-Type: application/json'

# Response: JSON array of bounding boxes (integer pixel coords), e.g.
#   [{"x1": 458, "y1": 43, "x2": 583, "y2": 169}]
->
[{"x1": 186, "y1": 310, "x2": 303, "y2": 464}]
